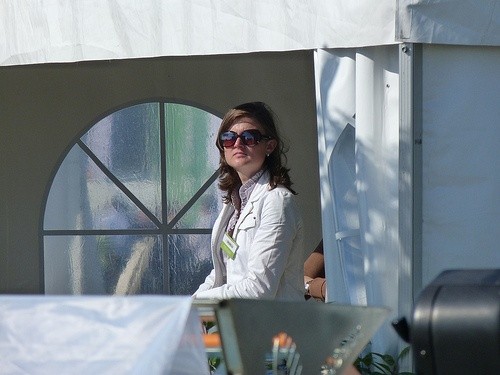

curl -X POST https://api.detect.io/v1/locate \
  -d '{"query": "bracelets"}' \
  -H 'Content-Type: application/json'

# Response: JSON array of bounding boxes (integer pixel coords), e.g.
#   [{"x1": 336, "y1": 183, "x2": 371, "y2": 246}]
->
[{"x1": 321, "y1": 280, "x2": 326, "y2": 299}]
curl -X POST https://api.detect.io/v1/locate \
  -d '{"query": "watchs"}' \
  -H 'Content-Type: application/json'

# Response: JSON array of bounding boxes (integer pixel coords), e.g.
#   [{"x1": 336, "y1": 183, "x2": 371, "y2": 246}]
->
[{"x1": 305, "y1": 279, "x2": 313, "y2": 296}]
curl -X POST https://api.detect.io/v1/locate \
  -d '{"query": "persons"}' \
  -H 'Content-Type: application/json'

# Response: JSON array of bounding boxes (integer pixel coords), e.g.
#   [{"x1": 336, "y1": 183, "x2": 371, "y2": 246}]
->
[
  {"x1": 191, "y1": 101, "x2": 306, "y2": 303},
  {"x1": 304, "y1": 237, "x2": 326, "y2": 299}
]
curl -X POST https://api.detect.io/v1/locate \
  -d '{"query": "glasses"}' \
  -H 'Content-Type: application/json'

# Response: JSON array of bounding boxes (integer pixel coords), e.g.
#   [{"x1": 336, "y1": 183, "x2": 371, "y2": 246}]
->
[{"x1": 219, "y1": 129, "x2": 271, "y2": 147}]
